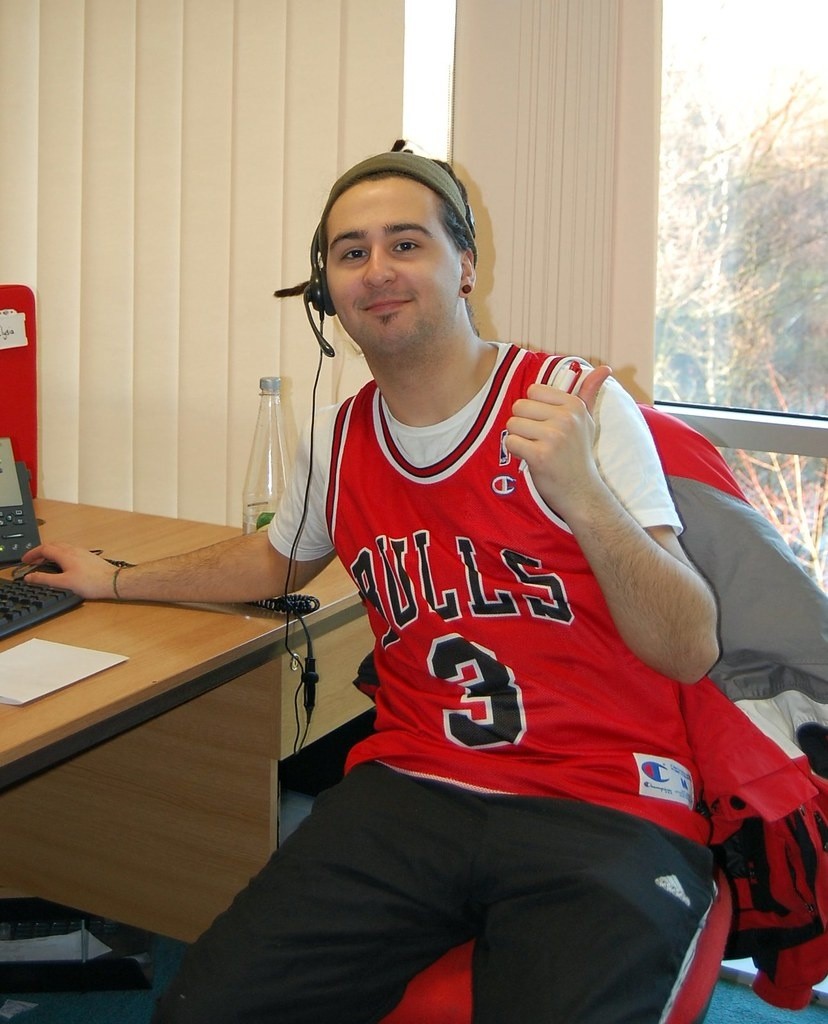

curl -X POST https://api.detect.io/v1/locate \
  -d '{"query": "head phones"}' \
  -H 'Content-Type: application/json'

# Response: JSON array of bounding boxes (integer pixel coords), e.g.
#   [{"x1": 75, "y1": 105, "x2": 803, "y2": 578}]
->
[{"x1": 305, "y1": 225, "x2": 336, "y2": 318}]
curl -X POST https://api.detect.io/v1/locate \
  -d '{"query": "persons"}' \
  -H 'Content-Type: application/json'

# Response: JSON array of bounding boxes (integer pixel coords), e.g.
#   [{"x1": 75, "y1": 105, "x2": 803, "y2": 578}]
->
[{"x1": 20, "y1": 153, "x2": 724, "y2": 1024}]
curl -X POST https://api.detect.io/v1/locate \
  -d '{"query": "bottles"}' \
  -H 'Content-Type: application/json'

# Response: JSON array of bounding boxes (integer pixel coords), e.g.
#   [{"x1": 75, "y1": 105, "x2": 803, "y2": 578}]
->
[{"x1": 243, "y1": 376, "x2": 291, "y2": 534}]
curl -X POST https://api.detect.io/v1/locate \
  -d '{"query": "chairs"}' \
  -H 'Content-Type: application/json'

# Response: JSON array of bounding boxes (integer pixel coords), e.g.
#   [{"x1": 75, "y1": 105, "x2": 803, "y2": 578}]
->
[{"x1": 377, "y1": 408, "x2": 735, "y2": 1024}]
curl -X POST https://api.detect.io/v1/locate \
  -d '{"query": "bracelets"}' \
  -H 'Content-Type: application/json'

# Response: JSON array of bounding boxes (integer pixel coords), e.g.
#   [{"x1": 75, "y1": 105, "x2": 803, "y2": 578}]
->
[{"x1": 113, "y1": 567, "x2": 122, "y2": 600}]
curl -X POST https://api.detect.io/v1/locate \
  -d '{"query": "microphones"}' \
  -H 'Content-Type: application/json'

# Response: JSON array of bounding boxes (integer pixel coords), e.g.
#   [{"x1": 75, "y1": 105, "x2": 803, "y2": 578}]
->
[{"x1": 303, "y1": 284, "x2": 335, "y2": 357}]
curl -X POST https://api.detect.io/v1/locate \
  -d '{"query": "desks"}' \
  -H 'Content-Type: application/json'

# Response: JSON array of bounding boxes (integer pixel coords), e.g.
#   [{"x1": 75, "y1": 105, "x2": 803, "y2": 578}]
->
[{"x1": 0, "y1": 497, "x2": 377, "y2": 945}]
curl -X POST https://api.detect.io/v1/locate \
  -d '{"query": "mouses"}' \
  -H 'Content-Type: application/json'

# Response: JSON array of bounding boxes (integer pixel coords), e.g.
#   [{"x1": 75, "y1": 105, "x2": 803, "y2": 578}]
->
[{"x1": 12, "y1": 560, "x2": 63, "y2": 581}]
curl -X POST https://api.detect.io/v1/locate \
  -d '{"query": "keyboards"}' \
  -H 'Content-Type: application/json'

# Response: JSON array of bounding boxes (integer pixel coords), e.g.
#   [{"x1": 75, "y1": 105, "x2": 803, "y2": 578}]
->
[{"x1": 0, "y1": 578, "x2": 85, "y2": 640}]
